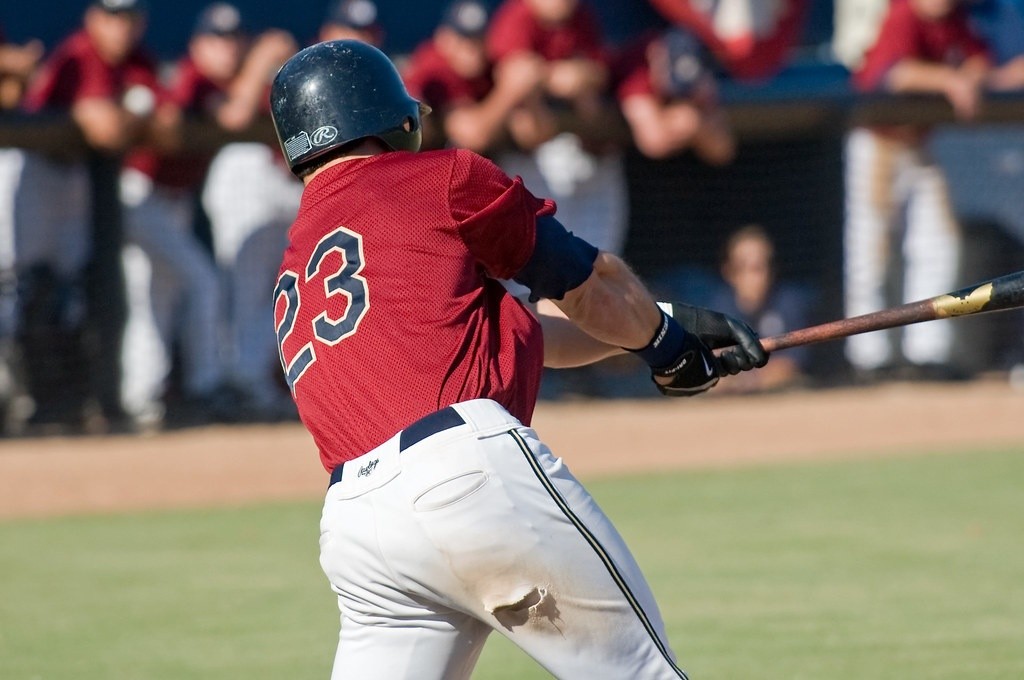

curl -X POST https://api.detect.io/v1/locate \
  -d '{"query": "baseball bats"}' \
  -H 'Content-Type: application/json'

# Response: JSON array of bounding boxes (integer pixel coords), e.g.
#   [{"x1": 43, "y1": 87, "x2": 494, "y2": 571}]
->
[{"x1": 654, "y1": 270, "x2": 1024, "y2": 386}]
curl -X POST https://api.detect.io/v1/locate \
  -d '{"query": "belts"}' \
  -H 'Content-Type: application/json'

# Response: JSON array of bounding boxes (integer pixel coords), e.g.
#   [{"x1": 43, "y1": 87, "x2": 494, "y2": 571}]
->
[{"x1": 327, "y1": 407, "x2": 466, "y2": 486}]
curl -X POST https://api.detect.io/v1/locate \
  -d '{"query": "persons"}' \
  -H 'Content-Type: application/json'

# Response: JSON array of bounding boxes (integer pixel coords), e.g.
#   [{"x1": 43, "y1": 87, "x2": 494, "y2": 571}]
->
[
  {"x1": 0, "y1": 0, "x2": 1024, "y2": 433},
  {"x1": 268, "y1": 39, "x2": 771, "y2": 680}
]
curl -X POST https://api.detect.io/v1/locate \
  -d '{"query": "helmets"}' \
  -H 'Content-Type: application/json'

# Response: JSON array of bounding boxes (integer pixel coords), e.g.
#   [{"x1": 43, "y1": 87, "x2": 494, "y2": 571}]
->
[{"x1": 269, "y1": 38, "x2": 433, "y2": 174}]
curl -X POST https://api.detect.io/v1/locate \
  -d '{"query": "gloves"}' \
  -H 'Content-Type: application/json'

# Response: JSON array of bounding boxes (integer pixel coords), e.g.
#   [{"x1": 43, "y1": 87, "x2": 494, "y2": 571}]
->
[
  {"x1": 671, "y1": 301, "x2": 769, "y2": 377},
  {"x1": 622, "y1": 304, "x2": 719, "y2": 396}
]
